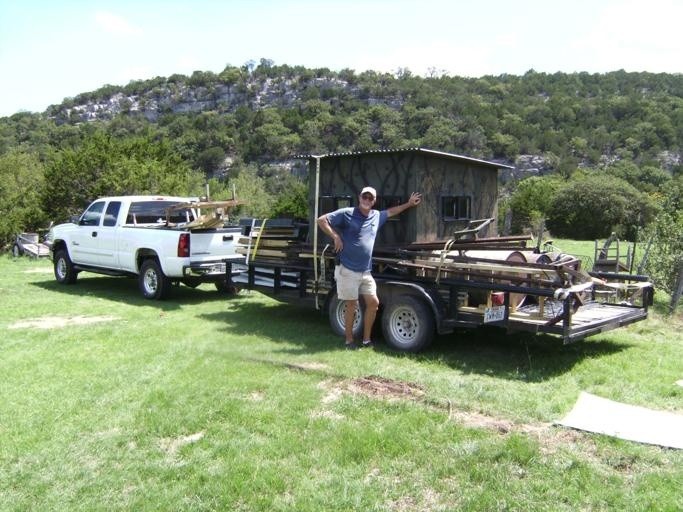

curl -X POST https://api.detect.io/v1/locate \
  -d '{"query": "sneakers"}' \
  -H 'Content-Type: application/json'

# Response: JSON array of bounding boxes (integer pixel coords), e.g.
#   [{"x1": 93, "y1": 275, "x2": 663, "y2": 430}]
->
[
  {"x1": 345, "y1": 343, "x2": 360, "y2": 350},
  {"x1": 360, "y1": 342, "x2": 375, "y2": 348}
]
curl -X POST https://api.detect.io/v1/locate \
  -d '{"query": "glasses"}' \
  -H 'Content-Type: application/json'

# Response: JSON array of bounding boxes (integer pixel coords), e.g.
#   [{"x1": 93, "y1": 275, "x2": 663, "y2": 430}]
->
[{"x1": 359, "y1": 195, "x2": 374, "y2": 201}]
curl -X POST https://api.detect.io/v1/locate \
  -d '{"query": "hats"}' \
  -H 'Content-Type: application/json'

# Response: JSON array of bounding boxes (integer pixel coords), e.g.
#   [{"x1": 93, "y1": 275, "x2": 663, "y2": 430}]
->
[{"x1": 361, "y1": 186, "x2": 377, "y2": 198}]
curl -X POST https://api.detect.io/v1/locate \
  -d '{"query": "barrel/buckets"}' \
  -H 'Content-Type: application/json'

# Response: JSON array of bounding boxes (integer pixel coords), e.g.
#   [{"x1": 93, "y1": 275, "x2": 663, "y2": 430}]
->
[
  {"x1": 20, "y1": 231, "x2": 39, "y2": 244},
  {"x1": 456, "y1": 292, "x2": 469, "y2": 309},
  {"x1": 462, "y1": 247, "x2": 575, "y2": 310},
  {"x1": 438, "y1": 284, "x2": 451, "y2": 310}
]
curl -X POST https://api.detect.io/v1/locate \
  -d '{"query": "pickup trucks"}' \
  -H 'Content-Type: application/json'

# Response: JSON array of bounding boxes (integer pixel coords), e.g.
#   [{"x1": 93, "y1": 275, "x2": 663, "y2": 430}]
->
[{"x1": 46, "y1": 195, "x2": 243, "y2": 300}]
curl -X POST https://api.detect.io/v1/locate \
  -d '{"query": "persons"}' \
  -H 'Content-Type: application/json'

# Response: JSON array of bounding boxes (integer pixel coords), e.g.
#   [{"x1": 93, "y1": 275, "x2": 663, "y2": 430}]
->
[{"x1": 316, "y1": 187, "x2": 422, "y2": 350}]
